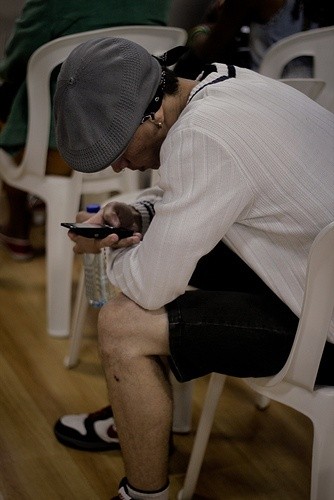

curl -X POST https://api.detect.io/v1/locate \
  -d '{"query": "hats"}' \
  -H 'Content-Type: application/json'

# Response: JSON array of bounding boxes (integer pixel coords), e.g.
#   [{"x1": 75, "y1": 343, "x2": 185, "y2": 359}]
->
[{"x1": 54, "y1": 37, "x2": 162, "y2": 174}]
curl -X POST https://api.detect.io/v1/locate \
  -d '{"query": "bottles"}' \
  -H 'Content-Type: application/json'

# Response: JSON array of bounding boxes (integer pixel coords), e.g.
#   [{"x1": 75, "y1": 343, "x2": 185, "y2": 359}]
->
[{"x1": 83, "y1": 204, "x2": 113, "y2": 307}]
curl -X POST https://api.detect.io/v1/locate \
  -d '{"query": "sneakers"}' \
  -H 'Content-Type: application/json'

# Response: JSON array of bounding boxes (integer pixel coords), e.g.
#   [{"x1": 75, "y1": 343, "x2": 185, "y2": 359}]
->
[
  {"x1": 32, "y1": 204, "x2": 46, "y2": 226},
  {"x1": 110, "y1": 476, "x2": 136, "y2": 500},
  {"x1": 53, "y1": 404, "x2": 175, "y2": 454},
  {"x1": 0, "y1": 225, "x2": 35, "y2": 261}
]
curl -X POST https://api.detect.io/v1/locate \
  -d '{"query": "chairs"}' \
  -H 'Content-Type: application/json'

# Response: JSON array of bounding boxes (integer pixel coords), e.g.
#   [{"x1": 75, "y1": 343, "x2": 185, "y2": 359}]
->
[{"x1": 0, "y1": 26, "x2": 334, "y2": 500}]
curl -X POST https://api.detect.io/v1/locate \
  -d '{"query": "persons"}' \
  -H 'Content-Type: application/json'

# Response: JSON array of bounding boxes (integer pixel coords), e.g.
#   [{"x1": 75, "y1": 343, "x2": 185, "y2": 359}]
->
[
  {"x1": 0, "y1": 0, "x2": 174, "y2": 262},
  {"x1": 54, "y1": 38, "x2": 333, "y2": 500},
  {"x1": 174, "y1": 0, "x2": 334, "y2": 80}
]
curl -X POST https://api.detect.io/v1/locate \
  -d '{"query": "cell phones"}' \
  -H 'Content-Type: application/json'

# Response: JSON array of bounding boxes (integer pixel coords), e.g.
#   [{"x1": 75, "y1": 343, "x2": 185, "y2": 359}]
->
[{"x1": 61, "y1": 223, "x2": 134, "y2": 239}]
